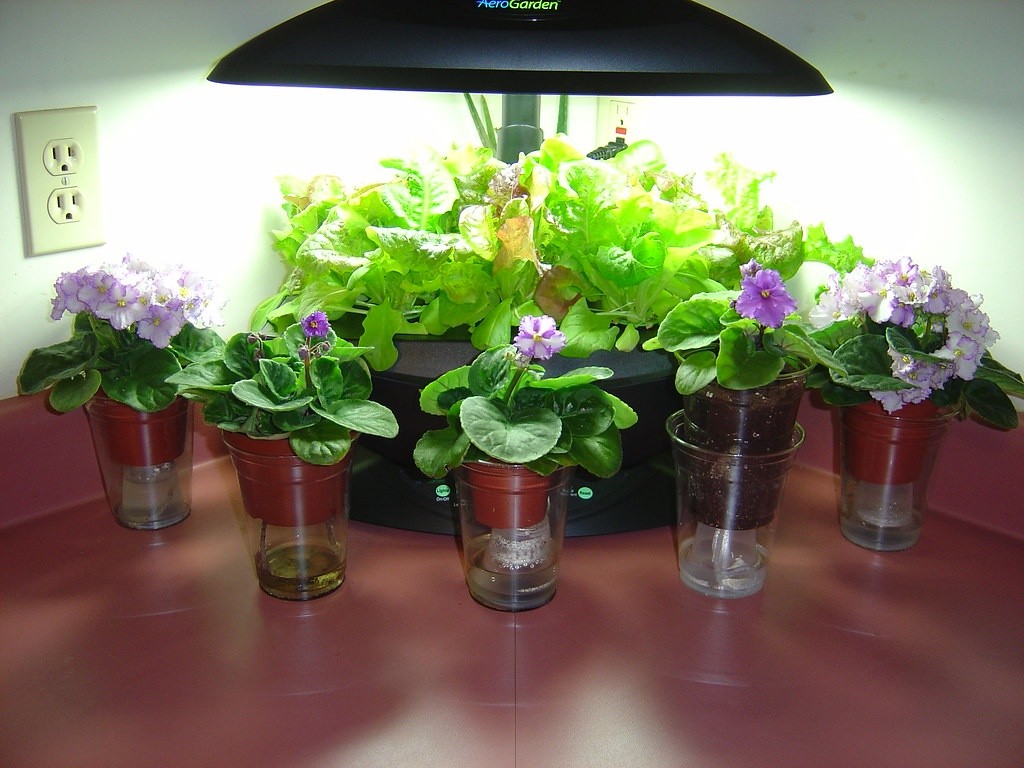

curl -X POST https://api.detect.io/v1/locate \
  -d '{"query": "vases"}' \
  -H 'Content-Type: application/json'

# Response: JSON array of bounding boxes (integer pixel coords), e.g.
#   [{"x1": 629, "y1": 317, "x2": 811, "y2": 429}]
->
[
  {"x1": 457, "y1": 459, "x2": 570, "y2": 611},
  {"x1": 665, "y1": 358, "x2": 813, "y2": 599},
  {"x1": 838, "y1": 394, "x2": 965, "y2": 554},
  {"x1": 218, "y1": 428, "x2": 361, "y2": 602},
  {"x1": 84, "y1": 387, "x2": 195, "y2": 530}
]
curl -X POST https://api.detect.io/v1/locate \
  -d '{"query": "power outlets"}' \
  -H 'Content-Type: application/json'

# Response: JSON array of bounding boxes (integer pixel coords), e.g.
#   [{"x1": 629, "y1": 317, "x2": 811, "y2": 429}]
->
[
  {"x1": 597, "y1": 97, "x2": 641, "y2": 148},
  {"x1": 16, "y1": 106, "x2": 110, "y2": 256}
]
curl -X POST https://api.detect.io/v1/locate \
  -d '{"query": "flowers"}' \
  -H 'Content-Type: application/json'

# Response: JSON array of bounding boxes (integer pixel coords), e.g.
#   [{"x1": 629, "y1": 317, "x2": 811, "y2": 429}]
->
[
  {"x1": 414, "y1": 314, "x2": 636, "y2": 478},
  {"x1": 804, "y1": 258, "x2": 1024, "y2": 431},
  {"x1": 14, "y1": 252, "x2": 227, "y2": 413},
  {"x1": 642, "y1": 259, "x2": 850, "y2": 394},
  {"x1": 165, "y1": 309, "x2": 399, "y2": 466}
]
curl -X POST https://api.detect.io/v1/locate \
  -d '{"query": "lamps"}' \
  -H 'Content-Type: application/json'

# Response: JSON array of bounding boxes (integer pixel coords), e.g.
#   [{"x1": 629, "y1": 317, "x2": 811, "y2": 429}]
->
[{"x1": 206, "y1": 0, "x2": 833, "y2": 96}]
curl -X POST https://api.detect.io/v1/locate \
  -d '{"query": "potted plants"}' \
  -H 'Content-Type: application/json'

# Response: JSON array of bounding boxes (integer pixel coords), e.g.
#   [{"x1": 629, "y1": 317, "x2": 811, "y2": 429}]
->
[{"x1": 248, "y1": 134, "x2": 874, "y2": 535}]
